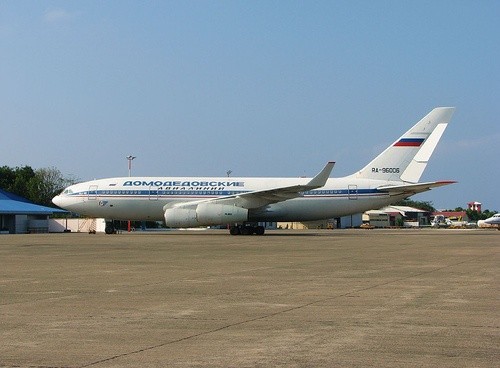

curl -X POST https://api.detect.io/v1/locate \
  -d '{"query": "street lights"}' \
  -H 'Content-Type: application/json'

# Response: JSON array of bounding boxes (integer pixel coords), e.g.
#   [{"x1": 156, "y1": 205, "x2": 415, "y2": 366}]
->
[{"x1": 124, "y1": 156, "x2": 136, "y2": 232}]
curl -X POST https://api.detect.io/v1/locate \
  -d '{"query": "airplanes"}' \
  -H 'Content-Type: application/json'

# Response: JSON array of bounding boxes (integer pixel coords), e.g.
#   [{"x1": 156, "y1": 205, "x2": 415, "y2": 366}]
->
[
  {"x1": 52, "y1": 106, "x2": 457, "y2": 235},
  {"x1": 484, "y1": 213, "x2": 500, "y2": 231}
]
